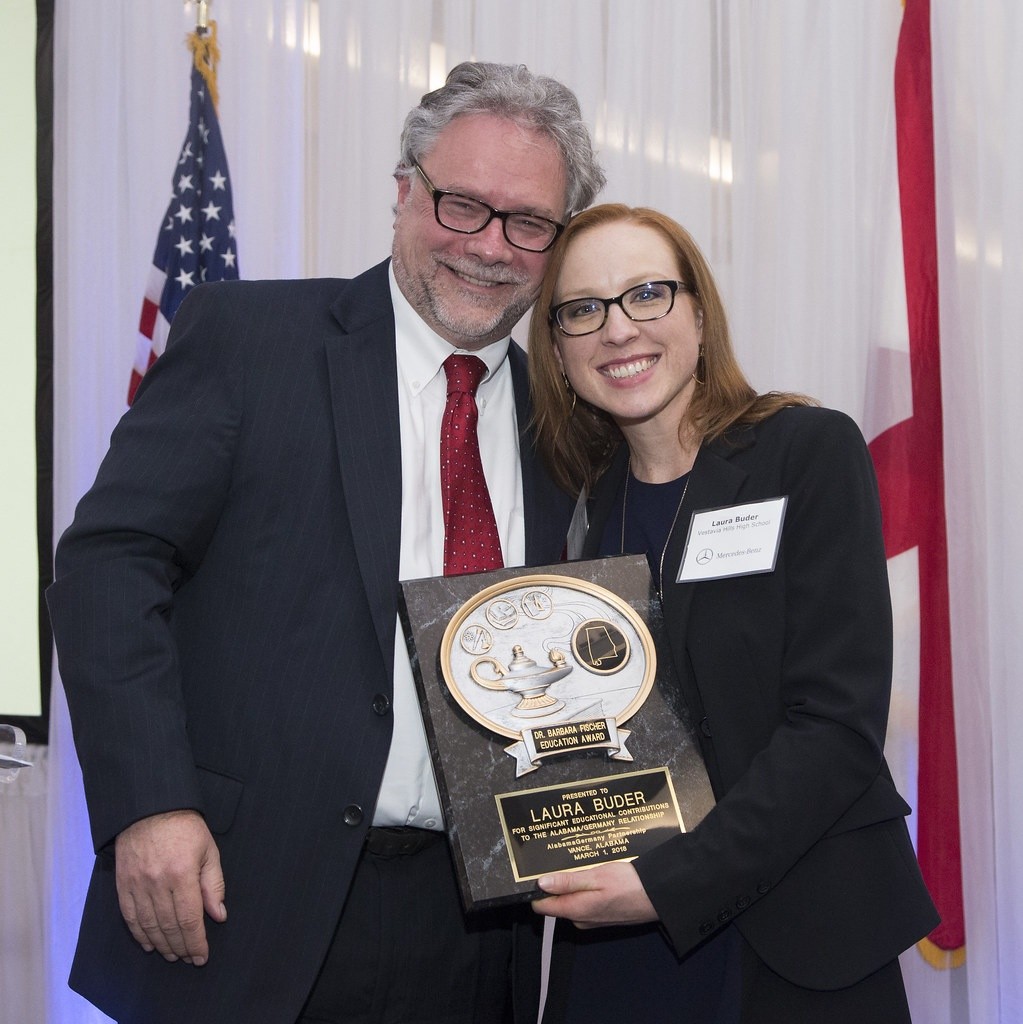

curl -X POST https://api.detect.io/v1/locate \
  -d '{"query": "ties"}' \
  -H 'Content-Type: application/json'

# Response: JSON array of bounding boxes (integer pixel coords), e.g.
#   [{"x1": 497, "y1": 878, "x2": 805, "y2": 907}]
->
[{"x1": 440, "y1": 354, "x2": 504, "y2": 573}]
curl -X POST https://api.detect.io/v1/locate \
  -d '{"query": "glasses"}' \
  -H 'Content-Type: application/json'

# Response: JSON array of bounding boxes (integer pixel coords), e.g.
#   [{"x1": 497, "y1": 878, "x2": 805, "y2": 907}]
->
[
  {"x1": 409, "y1": 150, "x2": 565, "y2": 252},
  {"x1": 547, "y1": 278, "x2": 692, "y2": 337}
]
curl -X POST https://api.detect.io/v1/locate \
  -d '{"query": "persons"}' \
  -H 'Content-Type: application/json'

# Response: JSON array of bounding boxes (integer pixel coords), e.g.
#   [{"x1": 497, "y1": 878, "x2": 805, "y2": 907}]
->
[
  {"x1": 45, "y1": 63, "x2": 605, "y2": 1024},
  {"x1": 529, "y1": 205, "x2": 942, "y2": 1024}
]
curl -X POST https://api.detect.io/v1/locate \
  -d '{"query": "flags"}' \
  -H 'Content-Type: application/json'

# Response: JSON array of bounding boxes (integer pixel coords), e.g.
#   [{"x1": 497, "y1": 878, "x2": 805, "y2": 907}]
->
[{"x1": 127, "y1": 20, "x2": 240, "y2": 405}]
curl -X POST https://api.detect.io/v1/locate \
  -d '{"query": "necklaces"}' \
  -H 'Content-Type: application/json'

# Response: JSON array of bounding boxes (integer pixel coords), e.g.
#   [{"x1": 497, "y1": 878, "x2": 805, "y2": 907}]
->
[{"x1": 621, "y1": 456, "x2": 691, "y2": 606}]
